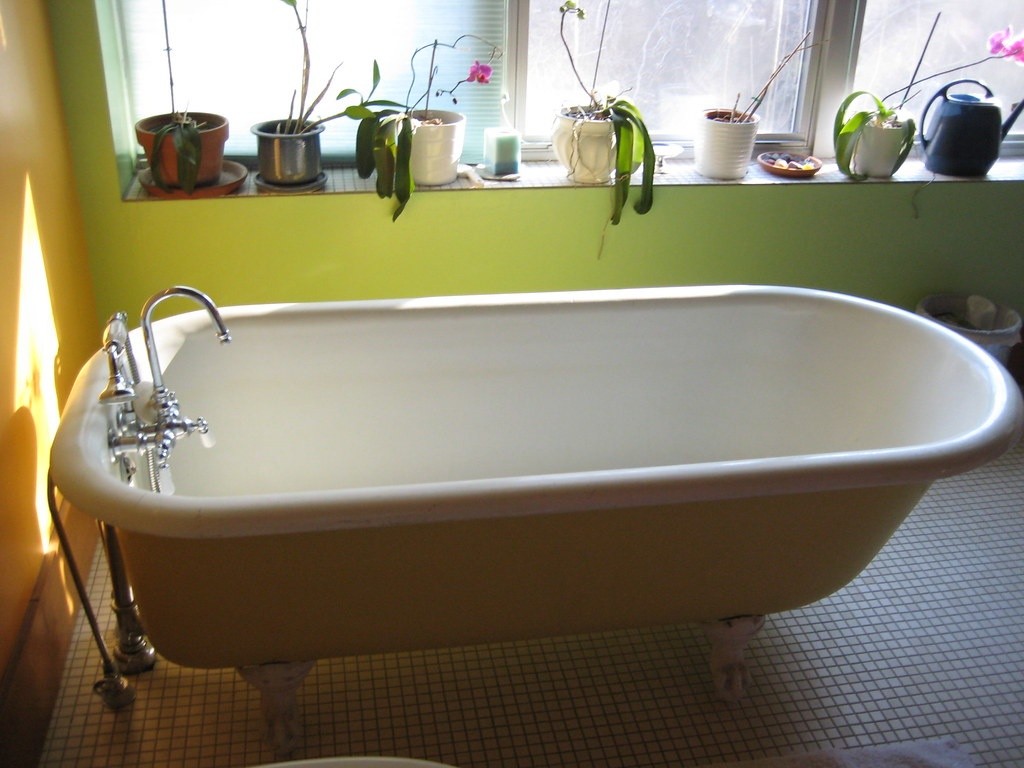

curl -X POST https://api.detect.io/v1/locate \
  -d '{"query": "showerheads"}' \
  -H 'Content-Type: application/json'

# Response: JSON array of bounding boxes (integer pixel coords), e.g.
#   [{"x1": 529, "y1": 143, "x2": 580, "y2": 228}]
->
[{"x1": 96, "y1": 374, "x2": 134, "y2": 407}]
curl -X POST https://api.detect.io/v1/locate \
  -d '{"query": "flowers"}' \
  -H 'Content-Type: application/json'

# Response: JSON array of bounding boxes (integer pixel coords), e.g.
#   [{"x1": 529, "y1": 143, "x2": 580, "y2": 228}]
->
[
  {"x1": 400, "y1": 33, "x2": 502, "y2": 119},
  {"x1": 834, "y1": 27, "x2": 1024, "y2": 180}
]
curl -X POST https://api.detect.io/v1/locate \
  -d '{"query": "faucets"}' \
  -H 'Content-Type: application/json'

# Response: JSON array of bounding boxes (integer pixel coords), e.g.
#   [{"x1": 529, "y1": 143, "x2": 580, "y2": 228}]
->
[{"x1": 138, "y1": 286, "x2": 233, "y2": 423}]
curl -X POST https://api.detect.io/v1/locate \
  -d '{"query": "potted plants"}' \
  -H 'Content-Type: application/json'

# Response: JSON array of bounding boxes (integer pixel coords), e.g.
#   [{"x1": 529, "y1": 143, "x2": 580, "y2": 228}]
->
[
  {"x1": 135, "y1": 103, "x2": 233, "y2": 196},
  {"x1": 248, "y1": 0, "x2": 414, "y2": 187},
  {"x1": 553, "y1": 1, "x2": 656, "y2": 224},
  {"x1": 695, "y1": 32, "x2": 831, "y2": 181}
]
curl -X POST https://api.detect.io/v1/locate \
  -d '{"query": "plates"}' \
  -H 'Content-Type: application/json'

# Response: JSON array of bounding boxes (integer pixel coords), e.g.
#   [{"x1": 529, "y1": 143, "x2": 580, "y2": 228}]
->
[{"x1": 757, "y1": 151, "x2": 822, "y2": 177}]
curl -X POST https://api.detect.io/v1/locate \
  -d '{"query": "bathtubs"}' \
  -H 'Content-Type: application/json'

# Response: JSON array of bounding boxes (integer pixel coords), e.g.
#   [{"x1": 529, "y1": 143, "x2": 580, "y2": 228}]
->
[{"x1": 50, "y1": 262, "x2": 1023, "y2": 672}]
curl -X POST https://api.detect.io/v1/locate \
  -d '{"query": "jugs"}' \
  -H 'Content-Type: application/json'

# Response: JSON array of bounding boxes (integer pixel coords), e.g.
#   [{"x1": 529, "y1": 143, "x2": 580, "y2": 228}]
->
[{"x1": 919, "y1": 78, "x2": 1024, "y2": 177}]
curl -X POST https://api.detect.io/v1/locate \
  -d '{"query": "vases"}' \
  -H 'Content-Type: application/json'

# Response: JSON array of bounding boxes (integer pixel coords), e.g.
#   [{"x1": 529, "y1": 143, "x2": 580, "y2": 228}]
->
[
  {"x1": 407, "y1": 110, "x2": 467, "y2": 185},
  {"x1": 851, "y1": 119, "x2": 916, "y2": 177}
]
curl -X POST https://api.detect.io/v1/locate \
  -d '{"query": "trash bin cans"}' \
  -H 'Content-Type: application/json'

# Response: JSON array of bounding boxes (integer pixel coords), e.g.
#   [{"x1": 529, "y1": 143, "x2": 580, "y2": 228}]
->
[{"x1": 916, "y1": 293, "x2": 1022, "y2": 369}]
